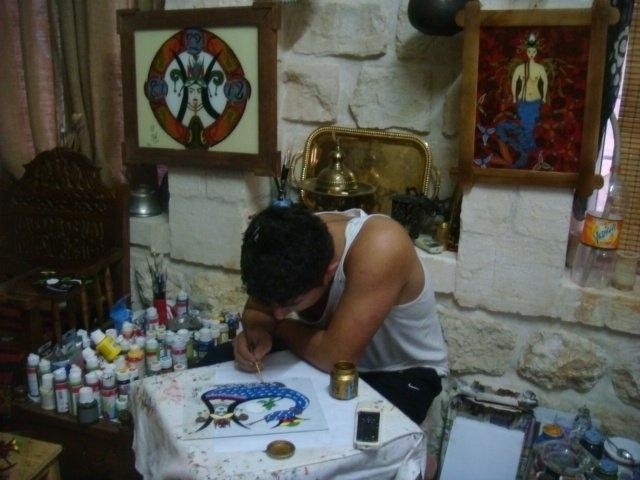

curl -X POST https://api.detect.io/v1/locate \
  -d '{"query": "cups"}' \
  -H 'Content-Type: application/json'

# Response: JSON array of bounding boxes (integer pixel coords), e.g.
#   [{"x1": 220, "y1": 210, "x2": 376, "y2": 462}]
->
[{"x1": 387, "y1": 197, "x2": 422, "y2": 239}]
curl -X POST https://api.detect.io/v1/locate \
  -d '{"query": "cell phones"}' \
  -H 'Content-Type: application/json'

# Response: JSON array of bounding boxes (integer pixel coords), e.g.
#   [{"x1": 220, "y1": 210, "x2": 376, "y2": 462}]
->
[{"x1": 353, "y1": 401, "x2": 383, "y2": 451}]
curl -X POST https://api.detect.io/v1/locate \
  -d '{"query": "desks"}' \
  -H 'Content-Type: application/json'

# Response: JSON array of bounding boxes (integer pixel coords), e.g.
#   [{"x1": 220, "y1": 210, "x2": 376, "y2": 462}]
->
[
  {"x1": 0, "y1": 431, "x2": 64, "y2": 480},
  {"x1": 128, "y1": 348, "x2": 429, "y2": 480}
]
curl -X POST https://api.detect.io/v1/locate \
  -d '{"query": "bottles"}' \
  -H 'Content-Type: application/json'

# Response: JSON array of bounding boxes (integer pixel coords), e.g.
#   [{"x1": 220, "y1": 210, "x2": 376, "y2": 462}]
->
[
  {"x1": 426, "y1": 213, "x2": 449, "y2": 246},
  {"x1": 589, "y1": 461, "x2": 620, "y2": 480},
  {"x1": 573, "y1": 406, "x2": 591, "y2": 436},
  {"x1": 568, "y1": 161, "x2": 633, "y2": 291},
  {"x1": 537, "y1": 423, "x2": 565, "y2": 442},
  {"x1": 583, "y1": 431, "x2": 604, "y2": 460},
  {"x1": 25, "y1": 289, "x2": 241, "y2": 424}
]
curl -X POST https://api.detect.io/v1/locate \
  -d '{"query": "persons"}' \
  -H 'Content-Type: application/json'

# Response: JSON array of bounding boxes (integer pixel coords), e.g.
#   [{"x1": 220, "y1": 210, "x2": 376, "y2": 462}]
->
[
  {"x1": 510, "y1": 38, "x2": 547, "y2": 167},
  {"x1": 231, "y1": 203, "x2": 450, "y2": 427}
]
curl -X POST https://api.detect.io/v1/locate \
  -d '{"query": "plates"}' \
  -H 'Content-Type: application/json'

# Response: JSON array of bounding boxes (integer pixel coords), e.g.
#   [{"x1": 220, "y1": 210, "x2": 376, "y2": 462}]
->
[
  {"x1": 47, "y1": 280, "x2": 82, "y2": 293},
  {"x1": 602, "y1": 434, "x2": 640, "y2": 465}
]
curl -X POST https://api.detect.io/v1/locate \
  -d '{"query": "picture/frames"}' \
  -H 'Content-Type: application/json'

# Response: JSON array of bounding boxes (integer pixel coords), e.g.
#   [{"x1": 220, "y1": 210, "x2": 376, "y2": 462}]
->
[
  {"x1": 112, "y1": 1, "x2": 283, "y2": 181},
  {"x1": 448, "y1": 0, "x2": 621, "y2": 197}
]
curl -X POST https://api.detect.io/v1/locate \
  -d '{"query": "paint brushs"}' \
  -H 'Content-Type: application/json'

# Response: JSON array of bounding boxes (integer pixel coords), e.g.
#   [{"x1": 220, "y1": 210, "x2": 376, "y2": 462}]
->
[
  {"x1": 237, "y1": 312, "x2": 264, "y2": 384},
  {"x1": 146, "y1": 252, "x2": 170, "y2": 299}
]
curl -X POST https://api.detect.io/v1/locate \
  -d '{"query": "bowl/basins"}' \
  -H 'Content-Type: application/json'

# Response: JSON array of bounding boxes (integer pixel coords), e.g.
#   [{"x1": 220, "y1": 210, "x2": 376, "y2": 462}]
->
[
  {"x1": 540, "y1": 440, "x2": 591, "y2": 478},
  {"x1": 40, "y1": 271, "x2": 56, "y2": 276},
  {"x1": 45, "y1": 278, "x2": 59, "y2": 285}
]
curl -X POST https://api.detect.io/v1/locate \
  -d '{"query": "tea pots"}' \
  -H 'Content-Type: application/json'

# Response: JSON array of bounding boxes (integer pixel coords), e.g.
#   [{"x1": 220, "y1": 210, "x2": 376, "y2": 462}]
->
[{"x1": 130, "y1": 183, "x2": 161, "y2": 218}]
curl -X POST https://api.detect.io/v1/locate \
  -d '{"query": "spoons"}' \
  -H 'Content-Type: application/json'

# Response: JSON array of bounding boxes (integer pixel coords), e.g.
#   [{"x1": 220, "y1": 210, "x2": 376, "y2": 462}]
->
[{"x1": 605, "y1": 435, "x2": 633, "y2": 460}]
